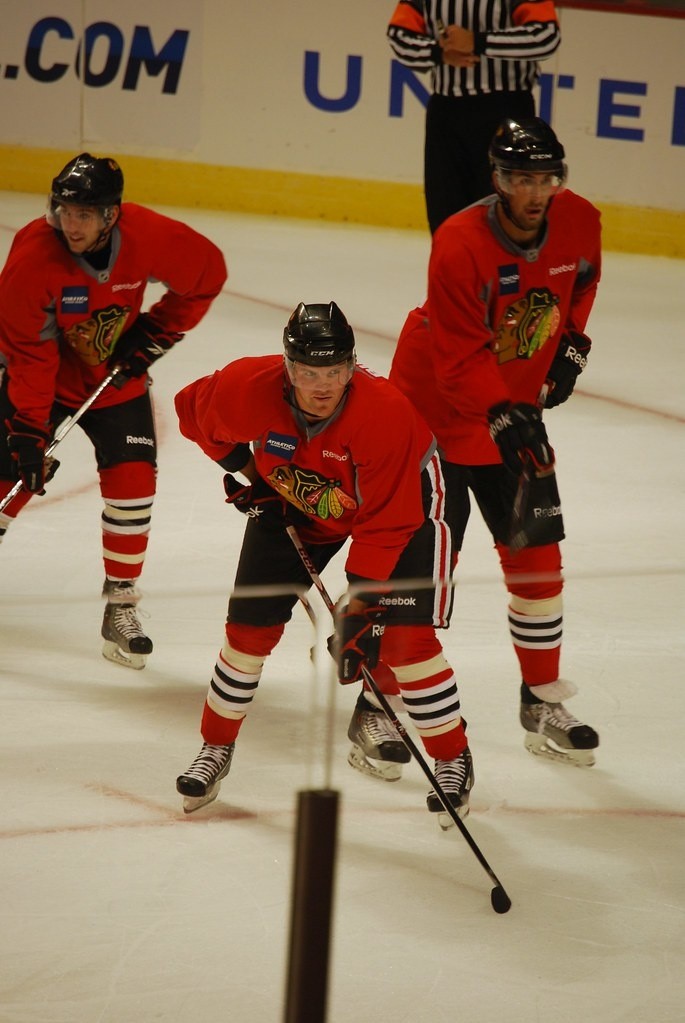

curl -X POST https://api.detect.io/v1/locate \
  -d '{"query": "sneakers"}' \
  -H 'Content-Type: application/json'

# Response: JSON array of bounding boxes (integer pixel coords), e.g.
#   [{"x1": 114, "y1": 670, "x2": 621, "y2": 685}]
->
[
  {"x1": 519, "y1": 677, "x2": 599, "y2": 768},
  {"x1": 176, "y1": 741, "x2": 234, "y2": 813},
  {"x1": 346, "y1": 690, "x2": 410, "y2": 782},
  {"x1": 101, "y1": 582, "x2": 154, "y2": 671},
  {"x1": 425, "y1": 746, "x2": 474, "y2": 830}
]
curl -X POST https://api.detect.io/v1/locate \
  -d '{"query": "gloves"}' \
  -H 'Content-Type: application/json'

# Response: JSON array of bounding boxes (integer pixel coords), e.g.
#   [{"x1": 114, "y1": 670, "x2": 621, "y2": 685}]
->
[
  {"x1": 115, "y1": 313, "x2": 185, "y2": 379},
  {"x1": 490, "y1": 403, "x2": 555, "y2": 485},
  {"x1": 544, "y1": 330, "x2": 592, "y2": 409},
  {"x1": 6, "y1": 413, "x2": 61, "y2": 496},
  {"x1": 326, "y1": 600, "x2": 387, "y2": 684},
  {"x1": 225, "y1": 473, "x2": 316, "y2": 533}
]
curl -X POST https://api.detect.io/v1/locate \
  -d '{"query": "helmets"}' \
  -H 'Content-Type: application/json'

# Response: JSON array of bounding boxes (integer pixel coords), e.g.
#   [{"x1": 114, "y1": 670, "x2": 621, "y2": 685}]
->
[
  {"x1": 488, "y1": 114, "x2": 565, "y2": 173},
  {"x1": 53, "y1": 154, "x2": 123, "y2": 207},
  {"x1": 282, "y1": 299, "x2": 355, "y2": 367}
]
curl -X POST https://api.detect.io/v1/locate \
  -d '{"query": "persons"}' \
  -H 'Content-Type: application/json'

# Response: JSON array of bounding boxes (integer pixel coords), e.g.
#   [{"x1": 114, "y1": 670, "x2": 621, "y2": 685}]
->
[
  {"x1": 0, "y1": 152, "x2": 228, "y2": 670},
  {"x1": 173, "y1": 302, "x2": 475, "y2": 831},
  {"x1": 385, "y1": 0, "x2": 562, "y2": 240},
  {"x1": 344, "y1": 115, "x2": 601, "y2": 780}
]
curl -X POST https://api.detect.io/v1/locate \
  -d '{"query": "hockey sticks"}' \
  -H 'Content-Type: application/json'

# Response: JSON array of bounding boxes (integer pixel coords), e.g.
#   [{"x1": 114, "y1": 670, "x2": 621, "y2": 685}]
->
[
  {"x1": 0, "y1": 363, "x2": 123, "y2": 515},
  {"x1": 284, "y1": 524, "x2": 512, "y2": 915},
  {"x1": 509, "y1": 383, "x2": 550, "y2": 552}
]
